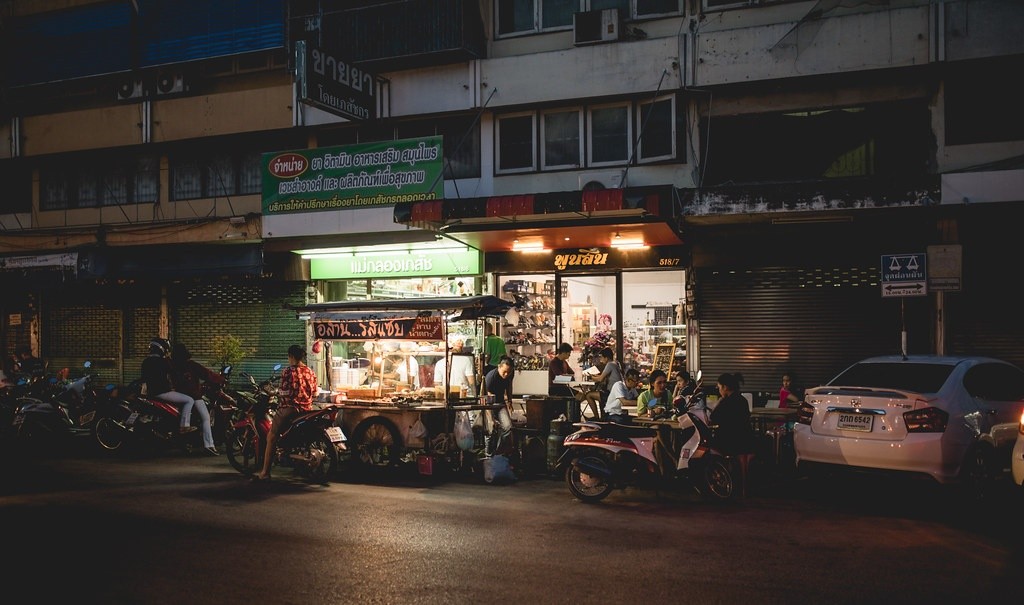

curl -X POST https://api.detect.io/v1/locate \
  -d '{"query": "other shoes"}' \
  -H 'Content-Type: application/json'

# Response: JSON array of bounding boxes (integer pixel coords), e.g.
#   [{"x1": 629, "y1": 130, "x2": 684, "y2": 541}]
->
[
  {"x1": 180, "y1": 426, "x2": 197, "y2": 434},
  {"x1": 206, "y1": 447, "x2": 220, "y2": 456},
  {"x1": 249, "y1": 474, "x2": 271, "y2": 483},
  {"x1": 588, "y1": 417, "x2": 598, "y2": 421}
]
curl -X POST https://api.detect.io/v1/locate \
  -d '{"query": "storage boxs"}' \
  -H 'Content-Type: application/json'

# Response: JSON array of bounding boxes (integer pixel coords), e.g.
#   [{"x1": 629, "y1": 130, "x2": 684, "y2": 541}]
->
[{"x1": 503, "y1": 279, "x2": 567, "y2": 297}]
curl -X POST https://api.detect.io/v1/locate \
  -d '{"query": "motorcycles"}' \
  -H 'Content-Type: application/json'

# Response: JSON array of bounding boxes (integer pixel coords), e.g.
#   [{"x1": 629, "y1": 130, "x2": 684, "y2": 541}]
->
[{"x1": 225, "y1": 363, "x2": 347, "y2": 483}]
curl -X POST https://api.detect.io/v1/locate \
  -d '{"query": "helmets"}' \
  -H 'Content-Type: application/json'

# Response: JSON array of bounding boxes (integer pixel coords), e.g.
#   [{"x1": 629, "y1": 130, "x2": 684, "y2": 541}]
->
[{"x1": 148, "y1": 338, "x2": 169, "y2": 357}]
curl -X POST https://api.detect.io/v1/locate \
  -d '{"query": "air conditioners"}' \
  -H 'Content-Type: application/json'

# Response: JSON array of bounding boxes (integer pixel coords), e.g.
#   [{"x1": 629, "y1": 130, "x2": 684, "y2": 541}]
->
[
  {"x1": 117, "y1": 77, "x2": 144, "y2": 100},
  {"x1": 579, "y1": 170, "x2": 627, "y2": 190},
  {"x1": 573, "y1": 8, "x2": 627, "y2": 47},
  {"x1": 157, "y1": 67, "x2": 185, "y2": 96}
]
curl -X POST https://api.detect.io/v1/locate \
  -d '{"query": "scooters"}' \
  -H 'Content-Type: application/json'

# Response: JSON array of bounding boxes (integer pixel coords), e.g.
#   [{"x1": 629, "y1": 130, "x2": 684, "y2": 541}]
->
[
  {"x1": 0, "y1": 357, "x2": 281, "y2": 457},
  {"x1": 562, "y1": 368, "x2": 737, "y2": 506}
]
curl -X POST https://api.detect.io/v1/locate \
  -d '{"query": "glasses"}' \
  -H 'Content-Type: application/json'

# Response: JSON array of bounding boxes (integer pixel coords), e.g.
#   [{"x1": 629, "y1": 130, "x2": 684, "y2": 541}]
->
[{"x1": 630, "y1": 379, "x2": 638, "y2": 384}]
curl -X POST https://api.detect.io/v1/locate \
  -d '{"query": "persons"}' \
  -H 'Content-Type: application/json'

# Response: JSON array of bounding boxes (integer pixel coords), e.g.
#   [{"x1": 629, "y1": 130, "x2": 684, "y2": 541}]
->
[
  {"x1": 412, "y1": 356, "x2": 437, "y2": 388},
  {"x1": 480, "y1": 355, "x2": 514, "y2": 458},
  {"x1": 485, "y1": 322, "x2": 507, "y2": 374},
  {"x1": 636, "y1": 370, "x2": 679, "y2": 474},
  {"x1": 671, "y1": 371, "x2": 697, "y2": 405},
  {"x1": 779, "y1": 373, "x2": 801, "y2": 409},
  {"x1": 141, "y1": 337, "x2": 198, "y2": 434},
  {"x1": 433, "y1": 339, "x2": 486, "y2": 480},
  {"x1": 703, "y1": 371, "x2": 751, "y2": 451},
  {"x1": 386, "y1": 351, "x2": 404, "y2": 365},
  {"x1": 170, "y1": 341, "x2": 226, "y2": 455},
  {"x1": 586, "y1": 348, "x2": 623, "y2": 421},
  {"x1": 248, "y1": 345, "x2": 317, "y2": 482},
  {"x1": 373, "y1": 348, "x2": 420, "y2": 389},
  {"x1": 4, "y1": 344, "x2": 38, "y2": 378},
  {"x1": 604, "y1": 369, "x2": 640, "y2": 424},
  {"x1": 548, "y1": 342, "x2": 586, "y2": 402}
]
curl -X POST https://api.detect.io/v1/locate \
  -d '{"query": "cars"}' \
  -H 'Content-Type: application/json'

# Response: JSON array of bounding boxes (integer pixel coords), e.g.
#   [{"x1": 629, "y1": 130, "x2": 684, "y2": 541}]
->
[{"x1": 793, "y1": 355, "x2": 1024, "y2": 502}]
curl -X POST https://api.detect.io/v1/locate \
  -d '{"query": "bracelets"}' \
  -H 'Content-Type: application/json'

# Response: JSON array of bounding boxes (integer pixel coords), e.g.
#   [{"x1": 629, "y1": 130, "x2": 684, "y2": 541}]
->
[{"x1": 485, "y1": 363, "x2": 488, "y2": 366}]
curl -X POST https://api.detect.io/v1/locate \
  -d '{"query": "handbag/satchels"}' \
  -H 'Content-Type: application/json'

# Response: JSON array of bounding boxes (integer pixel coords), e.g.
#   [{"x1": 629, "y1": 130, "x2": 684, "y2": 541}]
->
[
  {"x1": 484, "y1": 455, "x2": 517, "y2": 485},
  {"x1": 455, "y1": 412, "x2": 474, "y2": 451}
]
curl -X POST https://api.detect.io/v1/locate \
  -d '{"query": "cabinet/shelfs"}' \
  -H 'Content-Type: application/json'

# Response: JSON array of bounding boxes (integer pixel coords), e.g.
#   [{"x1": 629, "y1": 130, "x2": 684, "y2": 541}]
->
[{"x1": 503, "y1": 291, "x2": 566, "y2": 371}]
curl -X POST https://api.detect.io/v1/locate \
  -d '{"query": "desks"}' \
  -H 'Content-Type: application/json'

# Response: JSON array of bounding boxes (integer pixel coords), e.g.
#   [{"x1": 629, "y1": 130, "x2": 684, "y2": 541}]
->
[
  {"x1": 753, "y1": 408, "x2": 798, "y2": 437},
  {"x1": 553, "y1": 381, "x2": 598, "y2": 420},
  {"x1": 632, "y1": 417, "x2": 719, "y2": 452}
]
